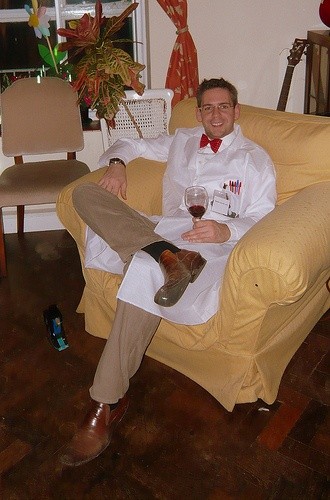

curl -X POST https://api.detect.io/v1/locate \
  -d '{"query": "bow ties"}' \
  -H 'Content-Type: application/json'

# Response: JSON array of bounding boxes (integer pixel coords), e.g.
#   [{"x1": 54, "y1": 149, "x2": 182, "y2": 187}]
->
[{"x1": 199, "y1": 133, "x2": 223, "y2": 153}]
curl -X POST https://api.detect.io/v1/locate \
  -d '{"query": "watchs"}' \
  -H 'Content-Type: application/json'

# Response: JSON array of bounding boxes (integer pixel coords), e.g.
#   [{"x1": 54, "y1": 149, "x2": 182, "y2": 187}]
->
[{"x1": 109, "y1": 158, "x2": 126, "y2": 167}]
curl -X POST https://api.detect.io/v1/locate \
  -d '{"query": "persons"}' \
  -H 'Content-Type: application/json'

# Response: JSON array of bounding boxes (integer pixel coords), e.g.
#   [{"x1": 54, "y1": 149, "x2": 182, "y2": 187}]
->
[{"x1": 58, "y1": 75, "x2": 277, "y2": 466}]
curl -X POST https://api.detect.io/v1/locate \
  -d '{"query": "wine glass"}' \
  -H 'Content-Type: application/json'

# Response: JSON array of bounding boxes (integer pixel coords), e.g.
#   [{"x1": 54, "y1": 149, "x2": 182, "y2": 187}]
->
[{"x1": 184, "y1": 185, "x2": 209, "y2": 222}]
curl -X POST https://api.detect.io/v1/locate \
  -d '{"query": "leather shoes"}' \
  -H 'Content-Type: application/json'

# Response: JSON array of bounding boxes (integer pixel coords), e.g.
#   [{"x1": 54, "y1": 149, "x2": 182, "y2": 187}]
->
[
  {"x1": 153, "y1": 248, "x2": 207, "y2": 307},
  {"x1": 60, "y1": 393, "x2": 130, "y2": 467}
]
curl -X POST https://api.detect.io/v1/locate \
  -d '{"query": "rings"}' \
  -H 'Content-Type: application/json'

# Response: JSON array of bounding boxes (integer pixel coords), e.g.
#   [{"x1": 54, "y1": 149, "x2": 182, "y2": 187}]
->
[{"x1": 106, "y1": 184, "x2": 113, "y2": 188}]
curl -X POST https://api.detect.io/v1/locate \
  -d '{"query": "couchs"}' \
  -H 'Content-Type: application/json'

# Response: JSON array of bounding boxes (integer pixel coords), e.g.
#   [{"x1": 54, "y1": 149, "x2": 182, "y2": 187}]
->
[{"x1": 56, "y1": 95, "x2": 330, "y2": 413}]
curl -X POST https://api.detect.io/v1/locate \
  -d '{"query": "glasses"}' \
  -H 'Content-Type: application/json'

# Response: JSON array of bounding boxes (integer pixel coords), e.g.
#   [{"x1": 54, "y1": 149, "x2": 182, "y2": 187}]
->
[{"x1": 196, "y1": 104, "x2": 235, "y2": 113}]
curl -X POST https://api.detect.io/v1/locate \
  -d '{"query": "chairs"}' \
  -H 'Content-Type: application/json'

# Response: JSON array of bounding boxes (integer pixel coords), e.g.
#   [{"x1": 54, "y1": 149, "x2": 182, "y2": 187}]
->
[
  {"x1": 99, "y1": 88, "x2": 175, "y2": 153},
  {"x1": 0, "y1": 77, "x2": 90, "y2": 279}
]
